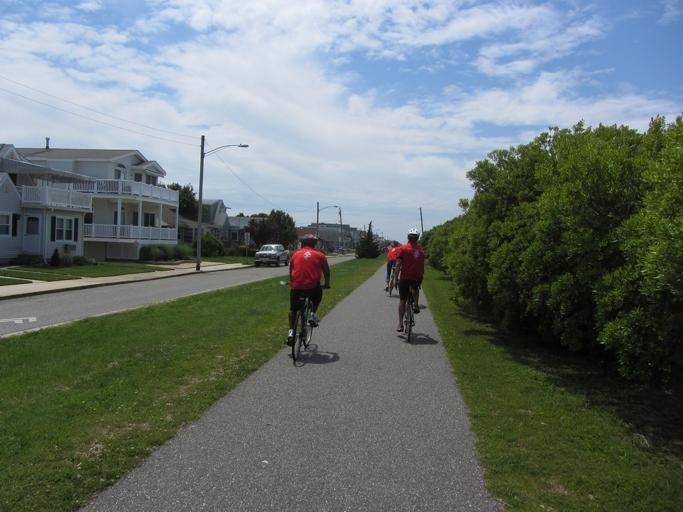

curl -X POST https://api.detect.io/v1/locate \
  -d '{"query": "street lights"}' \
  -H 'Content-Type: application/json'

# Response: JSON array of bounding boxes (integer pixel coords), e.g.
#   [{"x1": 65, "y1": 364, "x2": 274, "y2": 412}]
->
[
  {"x1": 195, "y1": 143, "x2": 250, "y2": 273},
  {"x1": 316, "y1": 205, "x2": 338, "y2": 238}
]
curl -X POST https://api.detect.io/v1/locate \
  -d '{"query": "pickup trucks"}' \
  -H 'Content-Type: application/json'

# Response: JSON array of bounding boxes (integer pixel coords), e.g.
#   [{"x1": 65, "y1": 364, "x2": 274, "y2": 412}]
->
[{"x1": 254, "y1": 244, "x2": 290, "y2": 267}]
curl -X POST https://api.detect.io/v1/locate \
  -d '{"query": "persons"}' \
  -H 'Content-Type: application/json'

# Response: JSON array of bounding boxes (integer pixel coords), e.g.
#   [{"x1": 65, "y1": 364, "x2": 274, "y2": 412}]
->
[
  {"x1": 392, "y1": 228, "x2": 425, "y2": 332},
  {"x1": 385, "y1": 241, "x2": 400, "y2": 290},
  {"x1": 287, "y1": 233, "x2": 330, "y2": 346}
]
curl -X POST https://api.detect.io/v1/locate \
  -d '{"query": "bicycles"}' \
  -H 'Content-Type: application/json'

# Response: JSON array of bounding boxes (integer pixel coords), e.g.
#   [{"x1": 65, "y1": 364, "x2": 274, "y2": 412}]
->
[
  {"x1": 279, "y1": 279, "x2": 332, "y2": 362},
  {"x1": 398, "y1": 284, "x2": 420, "y2": 343},
  {"x1": 386, "y1": 264, "x2": 398, "y2": 298}
]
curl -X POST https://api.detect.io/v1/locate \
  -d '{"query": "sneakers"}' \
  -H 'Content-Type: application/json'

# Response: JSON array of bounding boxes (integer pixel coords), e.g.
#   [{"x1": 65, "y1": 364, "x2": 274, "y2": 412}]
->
[
  {"x1": 413, "y1": 306, "x2": 419, "y2": 314},
  {"x1": 396, "y1": 326, "x2": 404, "y2": 332},
  {"x1": 287, "y1": 328, "x2": 295, "y2": 346},
  {"x1": 308, "y1": 317, "x2": 318, "y2": 327},
  {"x1": 384, "y1": 285, "x2": 388, "y2": 291}
]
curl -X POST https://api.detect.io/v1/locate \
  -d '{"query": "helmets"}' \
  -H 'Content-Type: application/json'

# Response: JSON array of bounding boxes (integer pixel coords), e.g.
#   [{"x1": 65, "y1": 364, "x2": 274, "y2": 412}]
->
[
  {"x1": 408, "y1": 228, "x2": 420, "y2": 235},
  {"x1": 299, "y1": 234, "x2": 318, "y2": 241}
]
